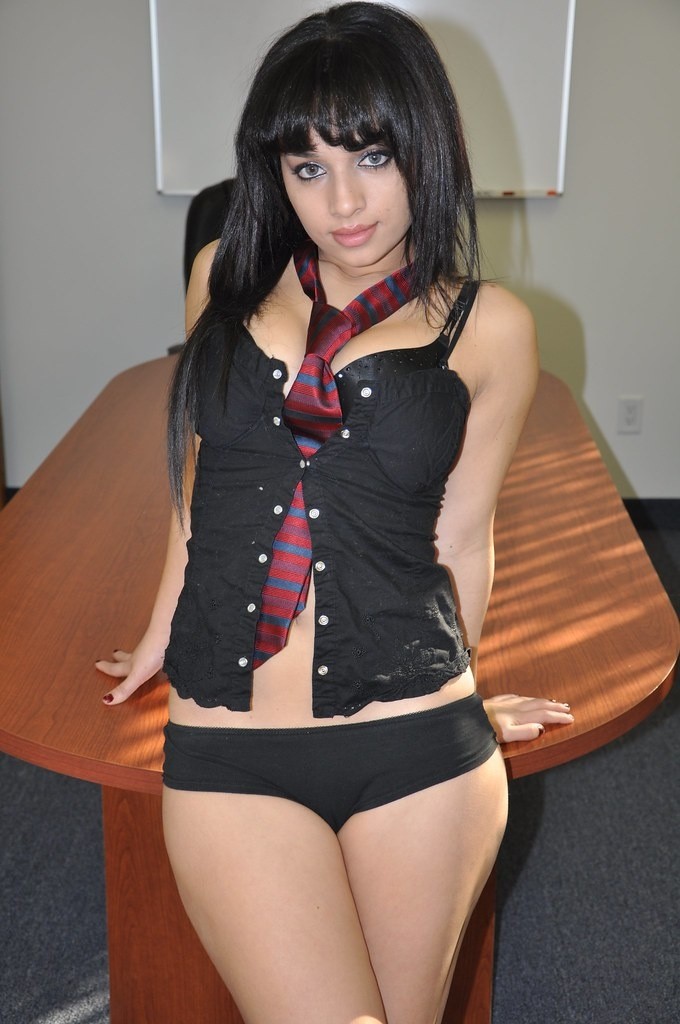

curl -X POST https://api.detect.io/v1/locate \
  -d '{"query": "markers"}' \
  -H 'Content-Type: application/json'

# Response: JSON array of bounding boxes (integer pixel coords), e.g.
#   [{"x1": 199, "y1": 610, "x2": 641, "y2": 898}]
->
[{"x1": 475, "y1": 190, "x2": 555, "y2": 198}]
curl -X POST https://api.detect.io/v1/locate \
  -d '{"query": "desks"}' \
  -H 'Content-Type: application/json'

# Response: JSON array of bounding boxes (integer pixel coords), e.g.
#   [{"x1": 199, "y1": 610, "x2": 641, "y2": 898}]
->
[{"x1": 0, "y1": 345, "x2": 680, "y2": 1024}]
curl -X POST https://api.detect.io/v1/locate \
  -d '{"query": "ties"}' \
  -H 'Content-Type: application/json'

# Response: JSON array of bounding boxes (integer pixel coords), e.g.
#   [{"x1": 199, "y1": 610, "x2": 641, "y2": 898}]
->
[{"x1": 246, "y1": 234, "x2": 437, "y2": 672}]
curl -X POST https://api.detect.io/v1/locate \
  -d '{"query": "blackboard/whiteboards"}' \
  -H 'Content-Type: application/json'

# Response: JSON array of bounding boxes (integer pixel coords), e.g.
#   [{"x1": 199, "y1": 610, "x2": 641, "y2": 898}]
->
[{"x1": 146, "y1": 0, "x2": 579, "y2": 204}]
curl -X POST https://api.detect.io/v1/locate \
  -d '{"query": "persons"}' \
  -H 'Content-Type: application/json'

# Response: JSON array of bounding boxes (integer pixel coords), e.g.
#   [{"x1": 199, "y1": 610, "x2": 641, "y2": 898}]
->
[{"x1": 94, "y1": 2, "x2": 575, "y2": 1024}]
